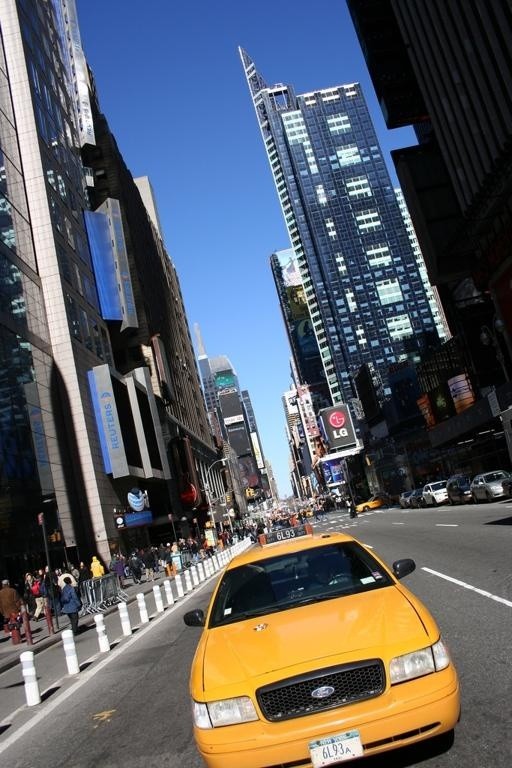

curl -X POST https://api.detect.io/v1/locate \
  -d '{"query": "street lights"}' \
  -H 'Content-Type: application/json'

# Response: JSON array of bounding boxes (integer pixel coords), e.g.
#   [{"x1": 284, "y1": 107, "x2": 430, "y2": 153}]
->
[{"x1": 204, "y1": 458, "x2": 229, "y2": 530}]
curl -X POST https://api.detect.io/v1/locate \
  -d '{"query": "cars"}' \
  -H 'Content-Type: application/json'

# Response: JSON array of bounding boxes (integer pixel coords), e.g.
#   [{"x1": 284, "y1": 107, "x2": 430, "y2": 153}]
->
[
  {"x1": 470, "y1": 469, "x2": 512, "y2": 503},
  {"x1": 446, "y1": 471, "x2": 479, "y2": 505},
  {"x1": 409, "y1": 487, "x2": 427, "y2": 508},
  {"x1": 301, "y1": 510, "x2": 314, "y2": 518},
  {"x1": 421, "y1": 479, "x2": 449, "y2": 508},
  {"x1": 181, "y1": 525, "x2": 461, "y2": 768},
  {"x1": 398, "y1": 490, "x2": 414, "y2": 508},
  {"x1": 354, "y1": 493, "x2": 400, "y2": 512}
]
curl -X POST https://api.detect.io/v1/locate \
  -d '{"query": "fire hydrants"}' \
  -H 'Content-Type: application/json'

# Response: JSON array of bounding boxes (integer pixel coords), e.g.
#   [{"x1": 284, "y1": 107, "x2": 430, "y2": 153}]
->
[{"x1": 5, "y1": 611, "x2": 23, "y2": 645}]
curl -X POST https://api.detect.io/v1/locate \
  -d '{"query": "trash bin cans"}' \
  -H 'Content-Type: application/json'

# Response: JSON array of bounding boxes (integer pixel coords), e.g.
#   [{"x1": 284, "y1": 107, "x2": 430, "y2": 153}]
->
[{"x1": 170, "y1": 551, "x2": 183, "y2": 573}]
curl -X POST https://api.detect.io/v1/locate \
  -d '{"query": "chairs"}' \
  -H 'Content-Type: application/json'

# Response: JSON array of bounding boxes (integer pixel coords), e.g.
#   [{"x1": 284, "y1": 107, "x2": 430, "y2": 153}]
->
[{"x1": 214, "y1": 545, "x2": 354, "y2": 612}]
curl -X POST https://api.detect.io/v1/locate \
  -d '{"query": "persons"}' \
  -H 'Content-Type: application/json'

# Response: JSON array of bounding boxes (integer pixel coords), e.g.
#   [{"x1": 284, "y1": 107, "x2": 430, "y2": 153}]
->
[
  {"x1": 1, "y1": 532, "x2": 215, "y2": 639},
  {"x1": 216, "y1": 487, "x2": 393, "y2": 549}
]
